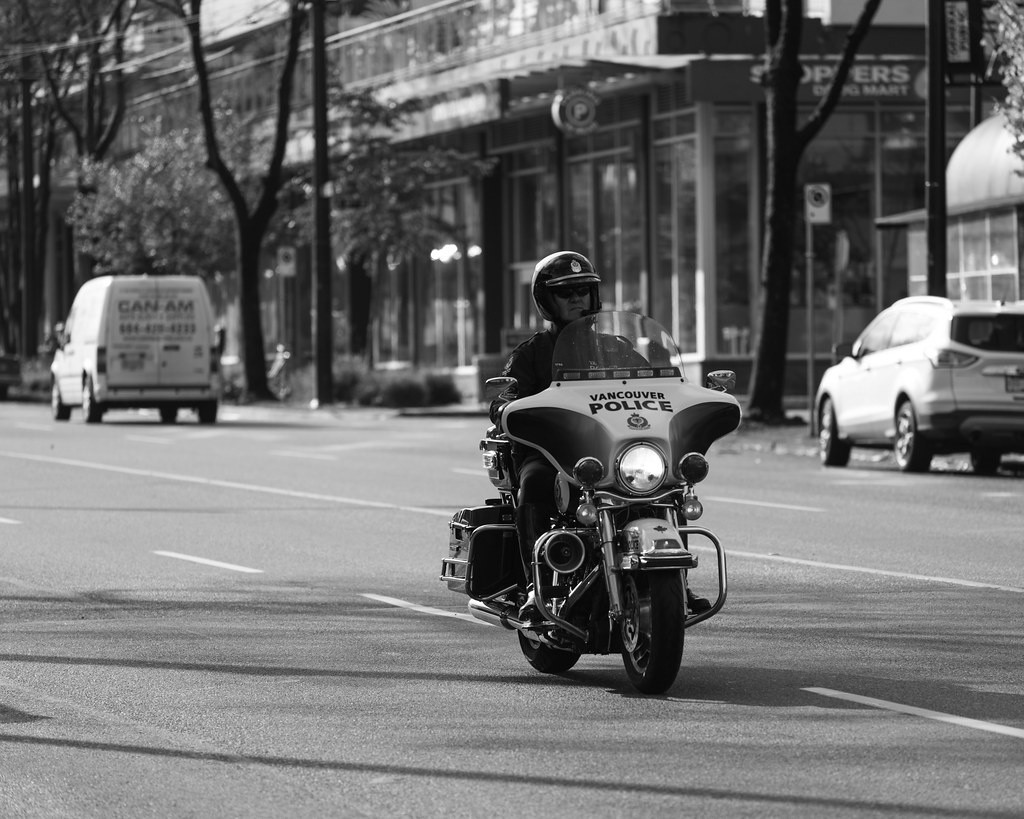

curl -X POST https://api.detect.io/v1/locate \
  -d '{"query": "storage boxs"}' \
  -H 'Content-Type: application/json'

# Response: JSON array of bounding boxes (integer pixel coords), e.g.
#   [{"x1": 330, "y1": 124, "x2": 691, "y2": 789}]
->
[{"x1": 446, "y1": 504, "x2": 515, "y2": 594}]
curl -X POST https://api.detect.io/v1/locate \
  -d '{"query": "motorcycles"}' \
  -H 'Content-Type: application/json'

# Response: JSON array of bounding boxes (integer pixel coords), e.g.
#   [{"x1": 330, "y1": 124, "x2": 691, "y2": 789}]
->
[{"x1": 439, "y1": 312, "x2": 743, "y2": 694}]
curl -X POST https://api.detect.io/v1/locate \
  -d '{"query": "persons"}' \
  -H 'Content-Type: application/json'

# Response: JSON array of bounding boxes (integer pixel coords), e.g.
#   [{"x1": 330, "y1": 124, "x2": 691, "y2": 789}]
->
[{"x1": 489, "y1": 251, "x2": 670, "y2": 621}]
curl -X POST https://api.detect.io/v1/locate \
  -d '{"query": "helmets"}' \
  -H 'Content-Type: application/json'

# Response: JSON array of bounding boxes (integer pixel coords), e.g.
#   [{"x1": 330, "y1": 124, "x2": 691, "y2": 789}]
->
[{"x1": 531, "y1": 251, "x2": 602, "y2": 322}]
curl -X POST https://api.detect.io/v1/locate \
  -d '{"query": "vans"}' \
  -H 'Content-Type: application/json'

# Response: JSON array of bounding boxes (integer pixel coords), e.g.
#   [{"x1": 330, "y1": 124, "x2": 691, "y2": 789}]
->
[{"x1": 50, "y1": 273, "x2": 221, "y2": 423}]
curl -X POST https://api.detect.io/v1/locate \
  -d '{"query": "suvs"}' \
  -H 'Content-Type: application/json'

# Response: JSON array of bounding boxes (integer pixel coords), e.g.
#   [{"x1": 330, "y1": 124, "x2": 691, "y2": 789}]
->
[{"x1": 814, "y1": 294, "x2": 1024, "y2": 474}]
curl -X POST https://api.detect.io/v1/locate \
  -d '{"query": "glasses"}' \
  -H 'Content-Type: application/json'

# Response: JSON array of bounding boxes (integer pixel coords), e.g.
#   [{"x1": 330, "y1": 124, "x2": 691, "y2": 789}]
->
[{"x1": 550, "y1": 286, "x2": 591, "y2": 299}]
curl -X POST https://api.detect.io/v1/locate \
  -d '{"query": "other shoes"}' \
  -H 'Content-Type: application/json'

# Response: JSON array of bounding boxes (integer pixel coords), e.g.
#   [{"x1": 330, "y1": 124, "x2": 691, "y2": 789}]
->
[
  {"x1": 518, "y1": 590, "x2": 543, "y2": 621},
  {"x1": 686, "y1": 587, "x2": 712, "y2": 614}
]
